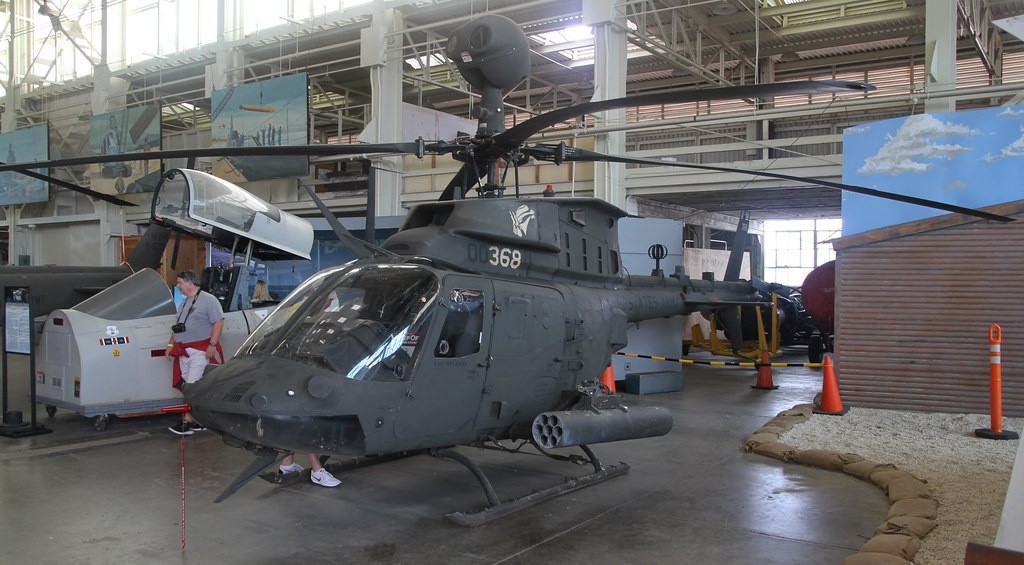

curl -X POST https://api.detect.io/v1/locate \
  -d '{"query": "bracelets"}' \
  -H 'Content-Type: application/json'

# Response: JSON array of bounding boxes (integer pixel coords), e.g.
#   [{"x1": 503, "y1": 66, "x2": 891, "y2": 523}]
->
[{"x1": 167, "y1": 344, "x2": 174, "y2": 347}]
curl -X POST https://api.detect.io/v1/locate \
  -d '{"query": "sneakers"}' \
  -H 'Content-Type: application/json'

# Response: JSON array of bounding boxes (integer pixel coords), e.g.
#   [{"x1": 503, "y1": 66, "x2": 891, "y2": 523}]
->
[
  {"x1": 279, "y1": 462, "x2": 304, "y2": 475},
  {"x1": 189, "y1": 424, "x2": 207, "y2": 431},
  {"x1": 310, "y1": 467, "x2": 343, "y2": 488},
  {"x1": 168, "y1": 421, "x2": 194, "y2": 436}
]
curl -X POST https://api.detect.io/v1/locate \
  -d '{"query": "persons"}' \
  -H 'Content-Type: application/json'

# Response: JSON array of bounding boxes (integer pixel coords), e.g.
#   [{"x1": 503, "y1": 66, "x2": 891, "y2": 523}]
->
[
  {"x1": 143, "y1": 137, "x2": 151, "y2": 175},
  {"x1": 240, "y1": 132, "x2": 245, "y2": 145},
  {"x1": 164, "y1": 269, "x2": 225, "y2": 435},
  {"x1": 251, "y1": 280, "x2": 274, "y2": 301},
  {"x1": 255, "y1": 130, "x2": 260, "y2": 143},
  {"x1": 272, "y1": 126, "x2": 275, "y2": 144},
  {"x1": 277, "y1": 434, "x2": 342, "y2": 487},
  {"x1": 277, "y1": 126, "x2": 283, "y2": 145},
  {"x1": 115, "y1": 173, "x2": 125, "y2": 195},
  {"x1": 267, "y1": 122, "x2": 271, "y2": 144},
  {"x1": 260, "y1": 125, "x2": 266, "y2": 145}
]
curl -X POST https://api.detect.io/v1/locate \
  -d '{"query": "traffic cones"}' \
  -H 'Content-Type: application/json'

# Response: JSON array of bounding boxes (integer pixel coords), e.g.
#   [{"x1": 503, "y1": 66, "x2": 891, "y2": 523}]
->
[
  {"x1": 751, "y1": 352, "x2": 781, "y2": 390},
  {"x1": 811, "y1": 356, "x2": 850, "y2": 416},
  {"x1": 601, "y1": 361, "x2": 617, "y2": 395}
]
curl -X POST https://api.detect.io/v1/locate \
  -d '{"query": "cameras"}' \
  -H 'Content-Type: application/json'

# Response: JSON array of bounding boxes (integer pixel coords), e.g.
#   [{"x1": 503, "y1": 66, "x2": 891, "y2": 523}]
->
[{"x1": 172, "y1": 323, "x2": 186, "y2": 333}]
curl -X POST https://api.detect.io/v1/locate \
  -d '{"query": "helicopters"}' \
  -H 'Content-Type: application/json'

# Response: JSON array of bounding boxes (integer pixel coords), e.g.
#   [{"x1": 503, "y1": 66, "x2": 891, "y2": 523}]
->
[
  {"x1": 0, "y1": 153, "x2": 198, "y2": 334},
  {"x1": 0, "y1": 13, "x2": 1020, "y2": 534},
  {"x1": 22, "y1": 168, "x2": 337, "y2": 430}
]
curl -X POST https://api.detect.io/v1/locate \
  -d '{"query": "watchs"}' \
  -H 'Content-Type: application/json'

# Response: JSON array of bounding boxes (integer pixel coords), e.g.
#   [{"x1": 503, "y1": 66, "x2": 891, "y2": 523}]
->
[{"x1": 209, "y1": 341, "x2": 217, "y2": 347}]
